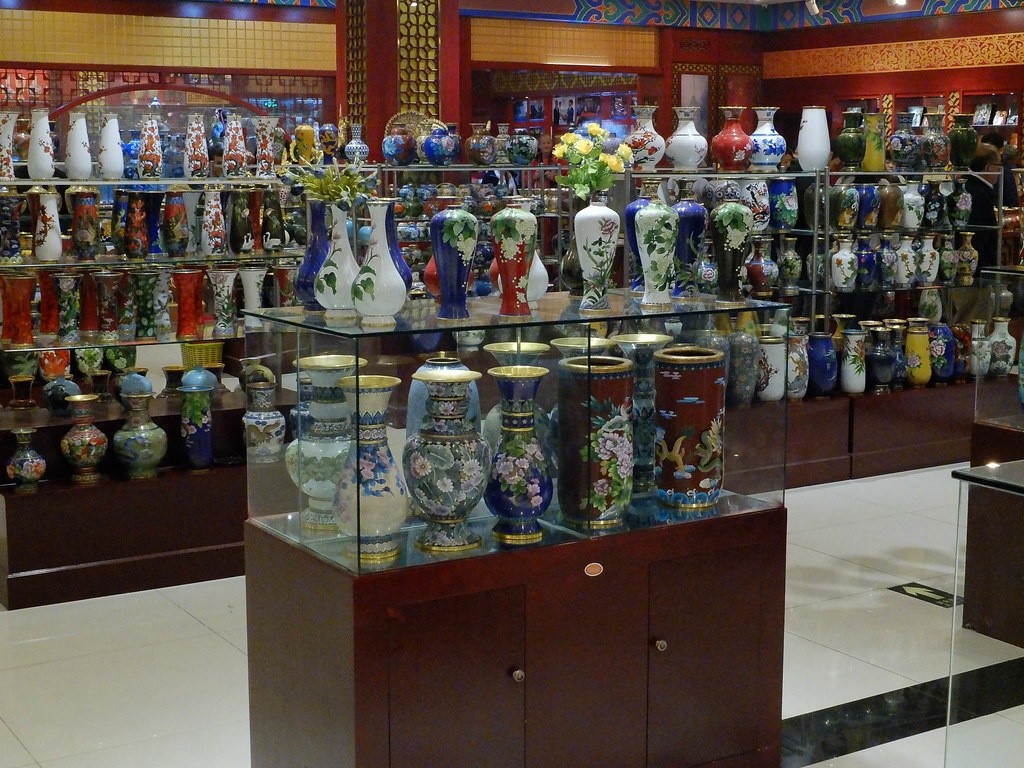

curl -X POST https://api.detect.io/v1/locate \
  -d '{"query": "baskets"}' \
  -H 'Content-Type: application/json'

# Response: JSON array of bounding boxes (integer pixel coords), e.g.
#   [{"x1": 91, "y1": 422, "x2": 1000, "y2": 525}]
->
[{"x1": 179, "y1": 342, "x2": 224, "y2": 369}]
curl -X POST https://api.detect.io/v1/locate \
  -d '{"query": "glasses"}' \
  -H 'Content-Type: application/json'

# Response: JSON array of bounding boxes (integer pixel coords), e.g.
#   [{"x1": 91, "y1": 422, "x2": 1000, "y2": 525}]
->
[{"x1": 990, "y1": 161, "x2": 1004, "y2": 166}]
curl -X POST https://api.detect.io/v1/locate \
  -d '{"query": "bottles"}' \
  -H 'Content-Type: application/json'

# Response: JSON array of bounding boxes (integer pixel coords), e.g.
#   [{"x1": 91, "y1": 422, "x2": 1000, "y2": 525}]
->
[{"x1": 1, "y1": 99, "x2": 1024, "y2": 564}]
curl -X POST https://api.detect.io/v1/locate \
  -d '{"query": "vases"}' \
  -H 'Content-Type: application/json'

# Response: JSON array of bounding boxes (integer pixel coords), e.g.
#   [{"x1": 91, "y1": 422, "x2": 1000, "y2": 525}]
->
[{"x1": 0, "y1": 97, "x2": 1024, "y2": 565}]
